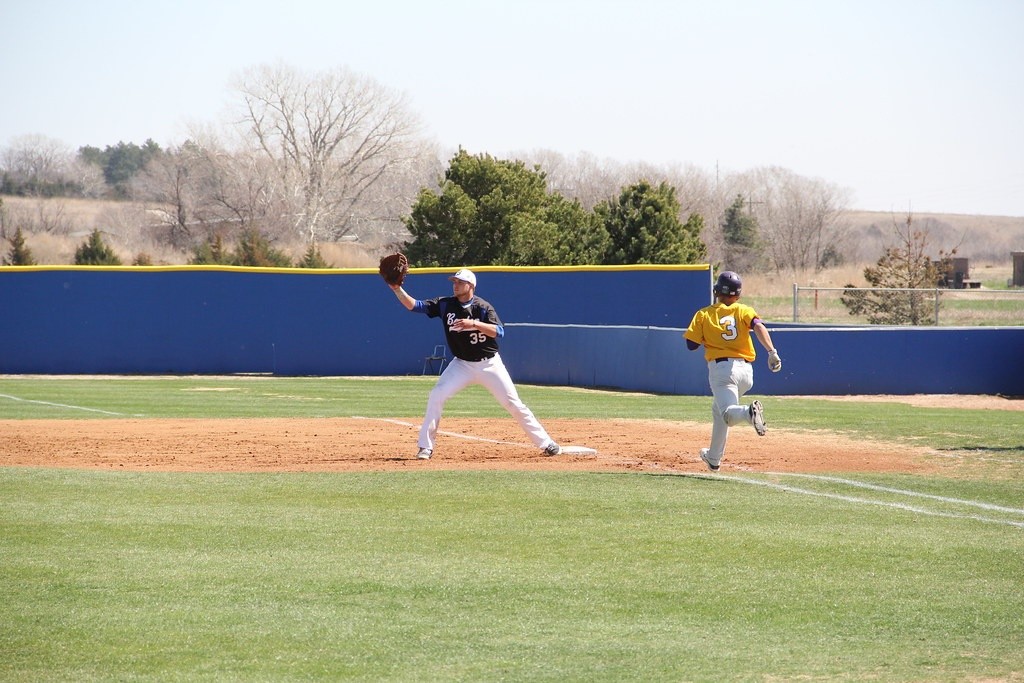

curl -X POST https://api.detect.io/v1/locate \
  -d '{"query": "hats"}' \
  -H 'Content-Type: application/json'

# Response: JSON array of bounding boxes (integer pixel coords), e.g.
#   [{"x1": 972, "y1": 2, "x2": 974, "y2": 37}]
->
[{"x1": 448, "y1": 269, "x2": 477, "y2": 289}]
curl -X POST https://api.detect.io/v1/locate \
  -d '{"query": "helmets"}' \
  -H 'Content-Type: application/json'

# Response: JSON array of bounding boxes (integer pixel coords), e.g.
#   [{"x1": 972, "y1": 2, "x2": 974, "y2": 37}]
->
[{"x1": 715, "y1": 271, "x2": 742, "y2": 297}]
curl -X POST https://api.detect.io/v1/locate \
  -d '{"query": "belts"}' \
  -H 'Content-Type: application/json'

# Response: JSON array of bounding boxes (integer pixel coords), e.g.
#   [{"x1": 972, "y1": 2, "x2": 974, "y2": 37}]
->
[{"x1": 715, "y1": 356, "x2": 749, "y2": 363}]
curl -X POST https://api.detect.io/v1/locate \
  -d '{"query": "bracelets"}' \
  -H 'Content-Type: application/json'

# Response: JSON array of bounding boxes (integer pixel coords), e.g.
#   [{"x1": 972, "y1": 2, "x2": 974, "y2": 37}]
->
[{"x1": 473, "y1": 320, "x2": 475, "y2": 327}]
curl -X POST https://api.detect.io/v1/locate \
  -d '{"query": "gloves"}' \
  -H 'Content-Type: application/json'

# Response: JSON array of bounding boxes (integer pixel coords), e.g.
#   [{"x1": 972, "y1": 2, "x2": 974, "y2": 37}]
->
[{"x1": 768, "y1": 348, "x2": 783, "y2": 373}]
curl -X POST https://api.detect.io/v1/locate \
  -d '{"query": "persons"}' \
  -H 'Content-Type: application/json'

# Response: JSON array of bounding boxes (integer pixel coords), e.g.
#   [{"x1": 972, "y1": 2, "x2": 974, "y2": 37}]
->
[
  {"x1": 682, "y1": 270, "x2": 782, "y2": 471},
  {"x1": 379, "y1": 253, "x2": 561, "y2": 460}
]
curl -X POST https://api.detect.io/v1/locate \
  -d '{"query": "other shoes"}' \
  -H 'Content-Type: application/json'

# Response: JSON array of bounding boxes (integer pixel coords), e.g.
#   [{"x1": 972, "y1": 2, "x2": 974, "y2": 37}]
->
[
  {"x1": 546, "y1": 441, "x2": 562, "y2": 456},
  {"x1": 748, "y1": 400, "x2": 767, "y2": 435},
  {"x1": 416, "y1": 448, "x2": 432, "y2": 460},
  {"x1": 700, "y1": 447, "x2": 719, "y2": 471}
]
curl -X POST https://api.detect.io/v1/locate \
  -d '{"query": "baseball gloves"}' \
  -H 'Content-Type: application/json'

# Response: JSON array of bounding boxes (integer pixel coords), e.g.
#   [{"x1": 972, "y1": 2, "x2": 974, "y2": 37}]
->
[{"x1": 379, "y1": 252, "x2": 409, "y2": 285}]
